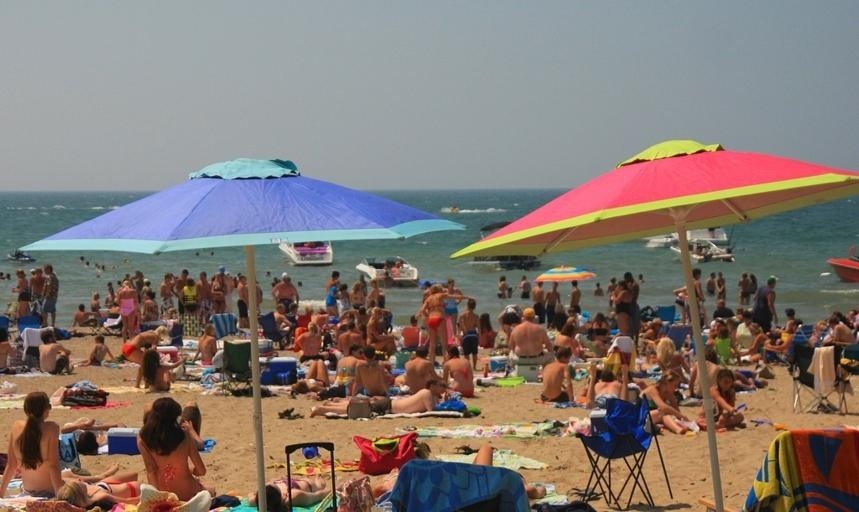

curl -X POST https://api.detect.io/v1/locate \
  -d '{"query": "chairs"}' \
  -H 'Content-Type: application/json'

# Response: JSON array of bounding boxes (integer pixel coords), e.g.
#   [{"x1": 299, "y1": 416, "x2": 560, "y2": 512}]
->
[
  {"x1": 699, "y1": 428, "x2": 859, "y2": 512},
  {"x1": 23, "y1": 328, "x2": 55, "y2": 360},
  {"x1": 575, "y1": 392, "x2": 674, "y2": 510},
  {"x1": 791, "y1": 347, "x2": 847, "y2": 414},
  {"x1": 210, "y1": 313, "x2": 237, "y2": 348},
  {"x1": 99, "y1": 314, "x2": 123, "y2": 338},
  {"x1": 222, "y1": 341, "x2": 251, "y2": 395},
  {"x1": 167, "y1": 320, "x2": 183, "y2": 361}
]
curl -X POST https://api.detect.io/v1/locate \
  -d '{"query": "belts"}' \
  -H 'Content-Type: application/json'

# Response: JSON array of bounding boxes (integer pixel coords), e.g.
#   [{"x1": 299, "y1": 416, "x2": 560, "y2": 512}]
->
[{"x1": 515, "y1": 352, "x2": 544, "y2": 359}]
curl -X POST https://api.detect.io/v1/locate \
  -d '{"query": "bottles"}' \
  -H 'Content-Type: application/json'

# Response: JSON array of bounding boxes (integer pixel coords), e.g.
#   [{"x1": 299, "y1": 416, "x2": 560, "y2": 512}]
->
[{"x1": 484, "y1": 363, "x2": 489, "y2": 378}]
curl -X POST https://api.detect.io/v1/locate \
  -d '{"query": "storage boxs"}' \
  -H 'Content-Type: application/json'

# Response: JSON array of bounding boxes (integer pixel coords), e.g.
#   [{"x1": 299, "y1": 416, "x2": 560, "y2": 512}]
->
[
  {"x1": 517, "y1": 364, "x2": 539, "y2": 382},
  {"x1": 107, "y1": 429, "x2": 138, "y2": 456},
  {"x1": 259, "y1": 356, "x2": 298, "y2": 384}
]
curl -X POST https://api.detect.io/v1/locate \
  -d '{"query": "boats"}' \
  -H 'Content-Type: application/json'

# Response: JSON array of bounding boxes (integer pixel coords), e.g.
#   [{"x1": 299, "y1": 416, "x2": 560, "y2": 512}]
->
[
  {"x1": 469, "y1": 221, "x2": 541, "y2": 271},
  {"x1": 279, "y1": 241, "x2": 334, "y2": 266},
  {"x1": 356, "y1": 254, "x2": 419, "y2": 286},
  {"x1": 826, "y1": 248, "x2": 859, "y2": 281},
  {"x1": 669, "y1": 239, "x2": 736, "y2": 263}
]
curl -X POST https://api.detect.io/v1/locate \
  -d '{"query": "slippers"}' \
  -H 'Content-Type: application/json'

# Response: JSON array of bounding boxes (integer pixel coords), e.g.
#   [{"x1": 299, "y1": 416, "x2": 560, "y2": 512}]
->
[
  {"x1": 567, "y1": 492, "x2": 598, "y2": 502},
  {"x1": 566, "y1": 486, "x2": 594, "y2": 495},
  {"x1": 448, "y1": 444, "x2": 479, "y2": 454}
]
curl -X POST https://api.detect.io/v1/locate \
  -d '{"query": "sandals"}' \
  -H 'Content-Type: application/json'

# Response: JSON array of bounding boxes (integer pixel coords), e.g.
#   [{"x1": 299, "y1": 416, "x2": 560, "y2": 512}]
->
[
  {"x1": 288, "y1": 414, "x2": 305, "y2": 420},
  {"x1": 278, "y1": 408, "x2": 294, "y2": 419}
]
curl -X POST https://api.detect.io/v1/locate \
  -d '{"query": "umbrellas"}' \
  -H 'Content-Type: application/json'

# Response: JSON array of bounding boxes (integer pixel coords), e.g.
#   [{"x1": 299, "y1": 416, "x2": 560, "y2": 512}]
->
[
  {"x1": 18, "y1": 155, "x2": 467, "y2": 512},
  {"x1": 446, "y1": 137, "x2": 859, "y2": 511}
]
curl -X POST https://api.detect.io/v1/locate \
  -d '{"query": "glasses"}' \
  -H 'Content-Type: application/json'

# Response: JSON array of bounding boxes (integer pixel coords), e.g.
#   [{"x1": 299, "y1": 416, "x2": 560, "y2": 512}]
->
[
  {"x1": 432, "y1": 384, "x2": 442, "y2": 387},
  {"x1": 44, "y1": 405, "x2": 52, "y2": 409}
]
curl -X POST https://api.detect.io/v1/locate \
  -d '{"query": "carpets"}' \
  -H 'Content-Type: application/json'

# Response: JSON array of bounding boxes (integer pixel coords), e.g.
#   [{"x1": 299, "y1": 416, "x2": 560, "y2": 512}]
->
[
  {"x1": 391, "y1": 419, "x2": 570, "y2": 442},
  {"x1": 324, "y1": 411, "x2": 465, "y2": 422},
  {"x1": 206, "y1": 492, "x2": 343, "y2": 512},
  {"x1": 0, "y1": 474, "x2": 49, "y2": 511},
  {"x1": 432, "y1": 449, "x2": 550, "y2": 472}
]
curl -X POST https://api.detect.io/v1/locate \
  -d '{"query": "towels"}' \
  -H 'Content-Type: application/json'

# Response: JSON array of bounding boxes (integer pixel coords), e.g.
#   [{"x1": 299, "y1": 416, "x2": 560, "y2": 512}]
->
[
  {"x1": 810, "y1": 346, "x2": 837, "y2": 396},
  {"x1": 390, "y1": 459, "x2": 531, "y2": 512}
]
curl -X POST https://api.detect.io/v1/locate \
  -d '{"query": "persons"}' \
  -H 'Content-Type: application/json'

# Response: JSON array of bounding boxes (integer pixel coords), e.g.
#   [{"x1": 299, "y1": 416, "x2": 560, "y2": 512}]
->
[
  {"x1": 473, "y1": 447, "x2": 546, "y2": 499},
  {"x1": 497, "y1": 272, "x2": 859, "y2": 437},
  {"x1": 0, "y1": 255, "x2": 495, "y2": 418},
  {"x1": 0, "y1": 392, "x2": 216, "y2": 512},
  {"x1": 247, "y1": 475, "x2": 343, "y2": 511}
]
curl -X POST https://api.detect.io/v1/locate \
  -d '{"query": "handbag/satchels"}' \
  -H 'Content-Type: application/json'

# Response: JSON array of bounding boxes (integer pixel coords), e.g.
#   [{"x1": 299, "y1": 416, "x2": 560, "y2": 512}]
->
[
  {"x1": 56, "y1": 433, "x2": 81, "y2": 471},
  {"x1": 347, "y1": 397, "x2": 372, "y2": 418},
  {"x1": 335, "y1": 476, "x2": 374, "y2": 512},
  {"x1": 352, "y1": 432, "x2": 420, "y2": 475}
]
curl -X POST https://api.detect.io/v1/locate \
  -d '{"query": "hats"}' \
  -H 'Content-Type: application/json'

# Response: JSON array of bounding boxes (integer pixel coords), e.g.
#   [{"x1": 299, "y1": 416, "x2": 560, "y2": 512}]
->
[
  {"x1": 523, "y1": 308, "x2": 535, "y2": 318},
  {"x1": 767, "y1": 274, "x2": 779, "y2": 284},
  {"x1": 282, "y1": 272, "x2": 287, "y2": 278},
  {"x1": 219, "y1": 265, "x2": 225, "y2": 272}
]
